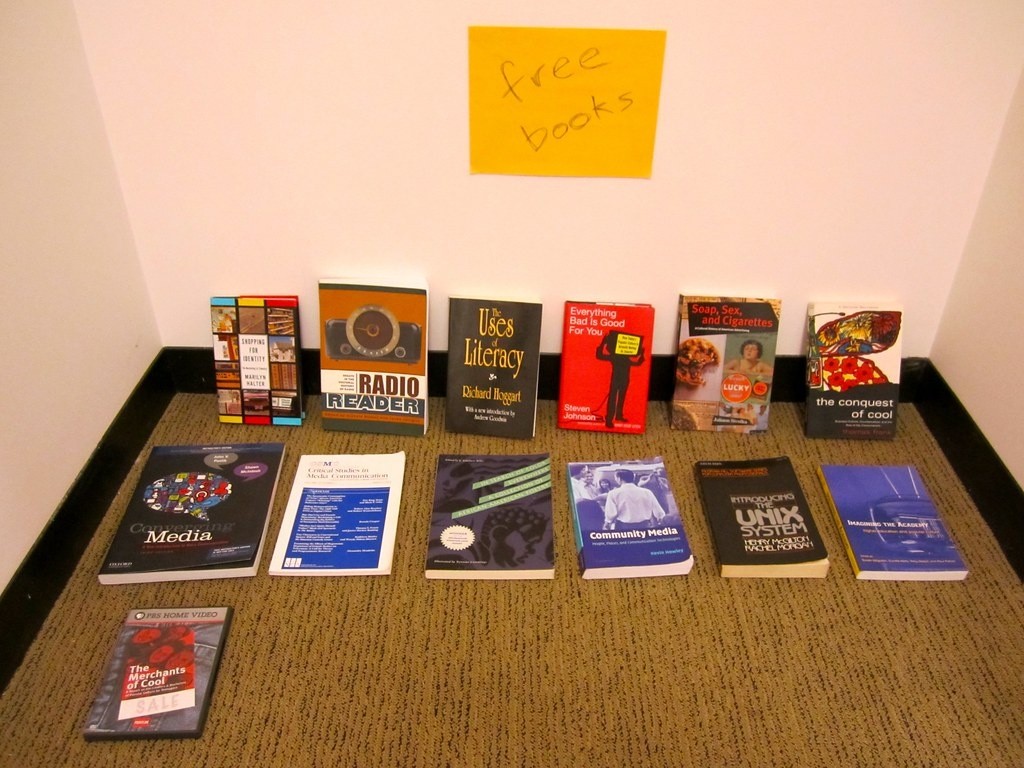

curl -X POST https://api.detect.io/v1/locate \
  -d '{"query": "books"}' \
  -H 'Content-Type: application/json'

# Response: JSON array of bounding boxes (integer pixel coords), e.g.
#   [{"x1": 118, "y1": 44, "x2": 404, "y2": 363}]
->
[
  {"x1": 695, "y1": 456, "x2": 830, "y2": 578},
  {"x1": 805, "y1": 301, "x2": 904, "y2": 440},
  {"x1": 424, "y1": 453, "x2": 556, "y2": 581},
  {"x1": 210, "y1": 295, "x2": 306, "y2": 427},
  {"x1": 557, "y1": 300, "x2": 655, "y2": 436},
  {"x1": 816, "y1": 465, "x2": 969, "y2": 580},
  {"x1": 567, "y1": 456, "x2": 694, "y2": 579},
  {"x1": 97, "y1": 441, "x2": 287, "y2": 585},
  {"x1": 317, "y1": 279, "x2": 430, "y2": 436},
  {"x1": 445, "y1": 296, "x2": 543, "y2": 439},
  {"x1": 669, "y1": 294, "x2": 781, "y2": 435},
  {"x1": 83, "y1": 605, "x2": 234, "y2": 742},
  {"x1": 268, "y1": 451, "x2": 407, "y2": 577}
]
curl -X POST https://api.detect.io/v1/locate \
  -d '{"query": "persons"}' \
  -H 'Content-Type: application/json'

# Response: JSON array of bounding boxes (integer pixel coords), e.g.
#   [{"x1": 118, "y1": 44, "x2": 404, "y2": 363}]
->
[
  {"x1": 130, "y1": 624, "x2": 195, "y2": 698},
  {"x1": 569, "y1": 464, "x2": 667, "y2": 533},
  {"x1": 723, "y1": 339, "x2": 772, "y2": 378}
]
